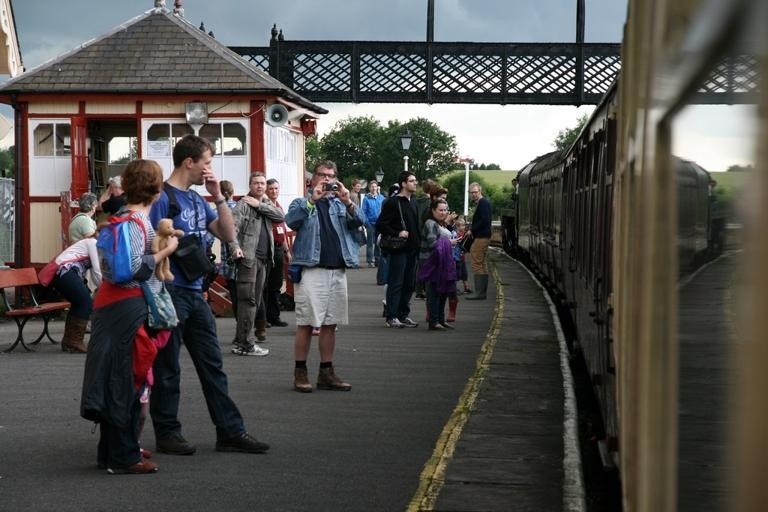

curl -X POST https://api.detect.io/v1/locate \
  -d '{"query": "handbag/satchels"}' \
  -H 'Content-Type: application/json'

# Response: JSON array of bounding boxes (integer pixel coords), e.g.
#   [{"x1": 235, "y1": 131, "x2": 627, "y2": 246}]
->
[
  {"x1": 170, "y1": 233, "x2": 215, "y2": 284},
  {"x1": 287, "y1": 264, "x2": 303, "y2": 283},
  {"x1": 378, "y1": 235, "x2": 407, "y2": 249},
  {"x1": 144, "y1": 287, "x2": 180, "y2": 330},
  {"x1": 37, "y1": 259, "x2": 61, "y2": 286},
  {"x1": 461, "y1": 233, "x2": 474, "y2": 252}
]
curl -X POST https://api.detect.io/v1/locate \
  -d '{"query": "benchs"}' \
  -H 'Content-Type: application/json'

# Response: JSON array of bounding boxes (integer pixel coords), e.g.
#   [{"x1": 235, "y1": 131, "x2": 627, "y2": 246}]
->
[{"x1": 0, "y1": 261, "x2": 75, "y2": 351}]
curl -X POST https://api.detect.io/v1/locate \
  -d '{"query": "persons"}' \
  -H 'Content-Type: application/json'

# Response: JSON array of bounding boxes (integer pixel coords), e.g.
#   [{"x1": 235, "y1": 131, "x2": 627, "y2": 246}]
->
[
  {"x1": 463, "y1": 182, "x2": 492, "y2": 301},
  {"x1": 362, "y1": 179, "x2": 385, "y2": 268},
  {"x1": 148, "y1": 134, "x2": 269, "y2": 452},
  {"x1": 132, "y1": 325, "x2": 173, "y2": 459},
  {"x1": 98, "y1": 173, "x2": 130, "y2": 216},
  {"x1": 286, "y1": 160, "x2": 367, "y2": 392},
  {"x1": 348, "y1": 178, "x2": 362, "y2": 208},
  {"x1": 51, "y1": 236, "x2": 102, "y2": 351},
  {"x1": 68, "y1": 191, "x2": 98, "y2": 335},
  {"x1": 375, "y1": 171, "x2": 474, "y2": 331},
  {"x1": 228, "y1": 171, "x2": 284, "y2": 357},
  {"x1": 210, "y1": 178, "x2": 267, "y2": 342},
  {"x1": 511, "y1": 179, "x2": 520, "y2": 196},
  {"x1": 82, "y1": 159, "x2": 179, "y2": 474},
  {"x1": 265, "y1": 181, "x2": 290, "y2": 329}
]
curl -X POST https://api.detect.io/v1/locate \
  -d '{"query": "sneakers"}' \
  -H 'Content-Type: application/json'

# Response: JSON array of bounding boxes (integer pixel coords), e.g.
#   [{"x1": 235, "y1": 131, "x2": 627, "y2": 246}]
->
[
  {"x1": 215, "y1": 432, "x2": 271, "y2": 453},
  {"x1": 161, "y1": 435, "x2": 197, "y2": 454},
  {"x1": 384, "y1": 318, "x2": 405, "y2": 329},
  {"x1": 414, "y1": 294, "x2": 427, "y2": 301},
  {"x1": 105, "y1": 458, "x2": 157, "y2": 475},
  {"x1": 273, "y1": 318, "x2": 288, "y2": 327},
  {"x1": 231, "y1": 344, "x2": 270, "y2": 356},
  {"x1": 138, "y1": 446, "x2": 154, "y2": 457},
  {"x1": 368, "y1": 262, "x2": 375, "y2": 268},
  {"x1": 255, "y1": 320, "x2": 271, "y2": 340},
  {"x1": 428, "y1": 322, "x2": 454, "y2": 331},
  {"x1": 464, "y1": 288, "x2": 473, "y2": 293},
  {"x1": 400, "y1": 317, "x2": 418, "y2": 327}
]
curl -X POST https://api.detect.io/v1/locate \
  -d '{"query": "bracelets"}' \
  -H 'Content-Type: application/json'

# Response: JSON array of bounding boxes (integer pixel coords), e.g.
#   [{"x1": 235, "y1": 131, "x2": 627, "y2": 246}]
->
[
  {"x1": 311, "y1": 196, "x2": 318, "y2": 202},
  {"x1": 344, "y1": 202, "x2": 354, "y2": 209}
]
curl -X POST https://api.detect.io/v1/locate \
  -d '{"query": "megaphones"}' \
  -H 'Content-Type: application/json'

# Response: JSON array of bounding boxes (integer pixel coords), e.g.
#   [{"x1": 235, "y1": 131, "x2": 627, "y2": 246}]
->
[{"x1": 267, "y1": 104, "x2": 289, "y2": 127}]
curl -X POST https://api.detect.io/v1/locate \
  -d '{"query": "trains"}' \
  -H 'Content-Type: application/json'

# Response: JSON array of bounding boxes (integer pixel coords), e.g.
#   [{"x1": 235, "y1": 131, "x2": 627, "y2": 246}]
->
[
  {"x1": 497, "y1": 2, "x2": 768, "y2": 510},
  {"x1": 672, "y1": 149, "x2": 732, "y2": 292}
]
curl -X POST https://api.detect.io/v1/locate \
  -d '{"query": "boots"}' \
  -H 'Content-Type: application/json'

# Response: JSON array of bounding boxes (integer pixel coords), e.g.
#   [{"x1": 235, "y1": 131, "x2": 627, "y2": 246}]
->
[
  {"x1": 315, "y1": 366, "x2": 352, "y2": 393},
  {"x1": 425, "y1": 303, "x2": 429, "y2": 321},
  {"x1": 382, "y1": 300, "x2": 387, "y2": 316},
  {"x1": 466, "y1": 274, "x2": 490, "y2": 300},
  {"x1": 292, "y1": 366, "x2": 313, "y2": 392},
  {"x1": 61, "y1": 314, "x2": 91, "y2": 353},
  {"x1": 447, "y1": 298, "x2": 460, "y2": 321}
]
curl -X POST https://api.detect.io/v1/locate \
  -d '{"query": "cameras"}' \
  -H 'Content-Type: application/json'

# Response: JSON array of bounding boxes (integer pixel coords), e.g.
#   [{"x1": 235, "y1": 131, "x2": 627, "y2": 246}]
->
[
  {"x1": 221, "y1": 256, "x2": 241, "y2": 281},
  {"x1": 326, "y1": 182, "x2": 339, "y2": 191}
]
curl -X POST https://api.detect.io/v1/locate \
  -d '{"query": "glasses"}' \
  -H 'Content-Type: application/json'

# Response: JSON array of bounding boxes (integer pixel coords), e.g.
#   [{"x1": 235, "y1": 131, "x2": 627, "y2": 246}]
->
[{"x1": 314, "y1": 172, "x2": 336, "y2": 179}]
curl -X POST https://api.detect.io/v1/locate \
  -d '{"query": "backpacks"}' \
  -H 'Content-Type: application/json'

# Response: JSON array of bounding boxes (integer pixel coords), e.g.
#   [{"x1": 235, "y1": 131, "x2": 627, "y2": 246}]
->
[{"x1": 96, "y1": 216, "x2": 134, "y2": 285}]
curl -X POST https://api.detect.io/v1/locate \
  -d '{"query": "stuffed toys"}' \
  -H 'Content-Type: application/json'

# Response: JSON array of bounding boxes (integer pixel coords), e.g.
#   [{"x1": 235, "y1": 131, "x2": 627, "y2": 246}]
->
[{"x1": 152, "y1": 215, "x2": 184, "y2": 283}]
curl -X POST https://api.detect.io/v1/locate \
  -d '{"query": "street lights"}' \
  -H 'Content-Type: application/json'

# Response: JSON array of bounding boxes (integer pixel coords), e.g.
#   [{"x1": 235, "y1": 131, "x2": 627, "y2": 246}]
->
[
  {"x1": 400, "y1": 126, "x2": 414, "y2": 177},
  {"x1": 374, "y1": 165, "x2": 385, "y2": 189}
]
